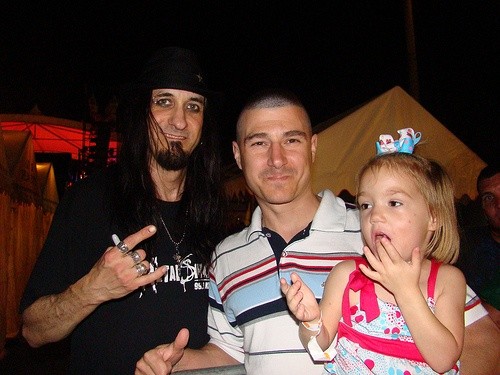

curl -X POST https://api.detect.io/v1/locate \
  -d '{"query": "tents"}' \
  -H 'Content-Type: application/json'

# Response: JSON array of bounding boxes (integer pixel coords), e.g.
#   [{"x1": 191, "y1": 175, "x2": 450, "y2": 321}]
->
[
  {"x1": 223, "y1": 86, "x2": 487, "y2": 239},
  {"x1": 0, "y1": 130, "x2": 46, "y2": 340},
  {"x1": 34, "y1": 162, "x2": 61, "y2": 258},
  {"x1": 0, "y1": 105, "x2": 123, "y2": 162}
]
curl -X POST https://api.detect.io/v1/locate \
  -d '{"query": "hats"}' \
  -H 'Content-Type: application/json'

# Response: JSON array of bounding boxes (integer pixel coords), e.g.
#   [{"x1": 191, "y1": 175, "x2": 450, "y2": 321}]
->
[{"x1": 135, "y1": 46, "x2": 210, "y2": 98}]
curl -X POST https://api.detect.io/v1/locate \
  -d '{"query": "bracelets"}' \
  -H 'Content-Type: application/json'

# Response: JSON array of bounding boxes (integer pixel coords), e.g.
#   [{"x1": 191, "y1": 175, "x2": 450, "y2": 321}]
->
[{"x1": 300, "y1": 306, "x2": 323, "y2": 332}]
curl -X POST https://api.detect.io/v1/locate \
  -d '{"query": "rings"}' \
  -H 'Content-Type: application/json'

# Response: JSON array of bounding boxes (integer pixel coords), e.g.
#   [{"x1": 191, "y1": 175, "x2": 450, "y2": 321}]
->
[
  {"x1": 117, "y1": 240, "x2": 130, "y2": 254},
  {"x1": 128, "y1": 250, "x2": 142, "y2": 264},
  {"x1": 134, "y1": 262, "x2": 149, "y2": 276}
]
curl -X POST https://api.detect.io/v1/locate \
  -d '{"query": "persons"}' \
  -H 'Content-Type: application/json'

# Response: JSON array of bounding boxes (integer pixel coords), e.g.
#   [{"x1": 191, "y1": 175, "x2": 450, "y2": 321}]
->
[
  {"x1": 134, "y1": 89, "x2": 500, "y2": 375},
  {"x1": 18, "y1": 48, "x2": 247, "y2": 375},
  {"x1": 280, "y1": 152, "x2": 465, "y2": 375},
  {"x1": 464, "y1": 163, "x2": 500, "y2": 331}
]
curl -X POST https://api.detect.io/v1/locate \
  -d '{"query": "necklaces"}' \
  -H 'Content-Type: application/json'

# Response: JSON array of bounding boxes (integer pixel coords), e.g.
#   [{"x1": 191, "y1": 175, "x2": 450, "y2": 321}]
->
[{"x1": 147, "y1": 193, "x2": 199, "y2": 269}]
